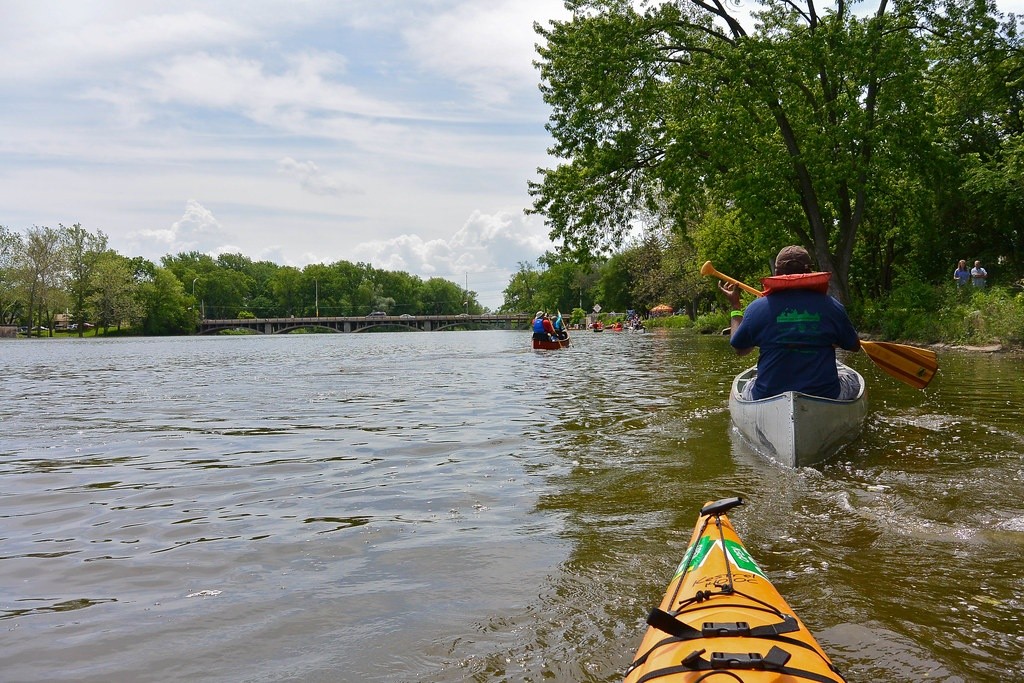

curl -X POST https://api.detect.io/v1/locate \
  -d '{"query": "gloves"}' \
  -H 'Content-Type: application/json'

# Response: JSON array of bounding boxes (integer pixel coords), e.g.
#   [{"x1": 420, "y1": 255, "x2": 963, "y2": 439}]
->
[{"x1": 551, "y1": 333, "x2": 558, "y2": 339}]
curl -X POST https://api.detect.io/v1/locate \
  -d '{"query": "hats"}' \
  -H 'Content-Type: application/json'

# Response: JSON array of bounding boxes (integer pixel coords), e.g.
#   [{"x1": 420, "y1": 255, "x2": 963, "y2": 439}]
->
[
  {"x1": 535, "y1": 311, "x2": 544, "y2": 319},
  {"x1": 774, "y1": 245, "x2": 815, "y2": 275}
]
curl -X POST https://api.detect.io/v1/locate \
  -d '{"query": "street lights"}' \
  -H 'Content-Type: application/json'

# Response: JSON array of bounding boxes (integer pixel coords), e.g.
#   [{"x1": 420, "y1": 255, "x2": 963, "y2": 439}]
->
[{"x1": 193, "y1": 278, "x2": 198, "y2": 306}]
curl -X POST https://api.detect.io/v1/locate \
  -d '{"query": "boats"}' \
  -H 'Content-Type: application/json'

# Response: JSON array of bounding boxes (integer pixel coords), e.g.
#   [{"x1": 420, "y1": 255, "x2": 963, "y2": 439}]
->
[
  {"x1": 628, "y1": 327, "x2": 645, "y2": 334},
  {"x1": 533, "y1": 330, "x2": 570, "y2": 350},
  {"x1": 729, "y1": 357, "x2": 868, "y2": 469},
  {"x1": 611, "y1": 326, "x2": 622, "y2": 331},
  {"x1": 593, "y1": 328, "x2": 603, "y2": 332}
]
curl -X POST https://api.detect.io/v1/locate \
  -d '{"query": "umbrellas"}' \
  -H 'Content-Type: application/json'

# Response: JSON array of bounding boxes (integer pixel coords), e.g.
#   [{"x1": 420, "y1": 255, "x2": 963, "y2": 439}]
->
[{"x1": 650, "y1": 305, "x2": 673, "y2": 313}]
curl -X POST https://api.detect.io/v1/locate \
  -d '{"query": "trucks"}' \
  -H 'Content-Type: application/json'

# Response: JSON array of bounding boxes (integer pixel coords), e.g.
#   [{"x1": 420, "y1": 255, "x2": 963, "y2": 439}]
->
[{"x1": 482, "y1": 312, "x2": 496, "y2": 318}]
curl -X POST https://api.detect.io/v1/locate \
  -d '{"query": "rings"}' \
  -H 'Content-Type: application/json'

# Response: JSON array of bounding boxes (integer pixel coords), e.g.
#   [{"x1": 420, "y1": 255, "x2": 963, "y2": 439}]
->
[{"x1": 718, "y1": 286, "x2": 721, "y2": 288}]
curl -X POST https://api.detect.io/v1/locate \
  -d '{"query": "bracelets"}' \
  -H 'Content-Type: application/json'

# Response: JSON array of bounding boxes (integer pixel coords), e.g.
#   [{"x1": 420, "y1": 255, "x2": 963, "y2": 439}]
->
[{"x1": 731, "y1": 310, "x2": 744, "y2": 316}]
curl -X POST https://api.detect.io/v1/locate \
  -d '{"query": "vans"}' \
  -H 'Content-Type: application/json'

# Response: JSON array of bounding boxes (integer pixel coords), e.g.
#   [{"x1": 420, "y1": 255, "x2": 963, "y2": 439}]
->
[{"x1": 366, "y1": 310, "x2": 386, "y2": 317}]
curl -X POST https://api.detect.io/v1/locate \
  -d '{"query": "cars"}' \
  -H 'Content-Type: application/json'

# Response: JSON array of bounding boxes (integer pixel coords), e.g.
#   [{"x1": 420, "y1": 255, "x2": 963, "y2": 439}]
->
[
  {"x1": 77, "y1": 323, "x2": 93, "y2": 329},
  {"x1": 455, "y1": 313, "x2": 471, "y2": 318},
  {"x1": 400, "y1": 313, "x2": 415, "y2": 318},
  {"x1": 31, "y1": 326, "x2": 48, "y2": 331}
]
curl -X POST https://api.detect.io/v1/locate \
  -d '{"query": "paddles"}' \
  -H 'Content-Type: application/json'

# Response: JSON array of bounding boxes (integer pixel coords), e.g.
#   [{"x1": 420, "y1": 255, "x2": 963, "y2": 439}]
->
[
  {"x1": 698, "y1": 259, "x2": 941, "y2": 391},
  {"x1": 547, "y1": 316, "x2": 563, "y2": 348},
  {"x1": 558, "y1": 309, "x2": 575, "y2": 349}
]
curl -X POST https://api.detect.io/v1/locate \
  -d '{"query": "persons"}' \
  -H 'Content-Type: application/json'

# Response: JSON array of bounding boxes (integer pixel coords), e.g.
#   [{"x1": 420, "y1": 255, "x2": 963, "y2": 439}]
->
[
  {"x1": 587, "y1": 320, "x2": 604, "y2": 331},
  {"x1": 574, "y1": 324, "x2": 583, "y2": 330},
  {"x1": 617, "y1": 322, "x2": 622, "y2": 328},
  {"x1": 645, "y1": 312, "x2": 658, "y2": 319},
  {"x1": 624, "y1": 314, "x2": 641, "y2": 331},
  {"x1": 566, "y1": 324, "x2": 568, "y2": 330},
  {"x1": 954, "y1": 260, "x2": 971, "y2": 285},
  {"x1": 531, "y1": 311, "x2": 565, "y2": 340},
  {"x1": 717, "y1": 246, "x2": 860, "y2": 399},
  {"x1": 971, "y1": 261, "x2": 987, "y2": 285}
]
